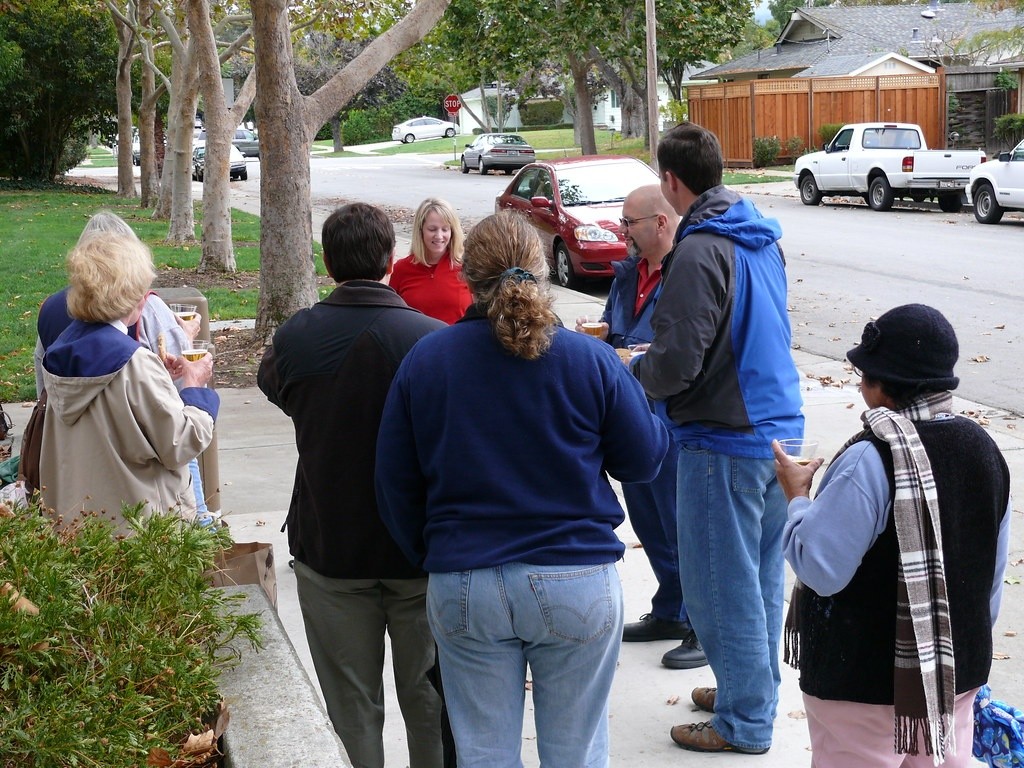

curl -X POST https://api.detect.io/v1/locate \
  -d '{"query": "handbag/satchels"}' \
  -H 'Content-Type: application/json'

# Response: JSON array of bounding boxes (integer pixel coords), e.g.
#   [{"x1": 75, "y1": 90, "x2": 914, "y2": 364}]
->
[{"x1": 18, "y1": 387, "x2": 47, "y2": 496}]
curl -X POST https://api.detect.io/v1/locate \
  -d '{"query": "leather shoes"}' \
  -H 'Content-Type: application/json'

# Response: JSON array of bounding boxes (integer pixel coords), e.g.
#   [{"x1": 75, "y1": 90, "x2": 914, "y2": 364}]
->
[
  {"x1": 621, "y1": 612, "x2": 691, "y2": 642},
  {"x1": 660, "y1": 630, "x2": 709, "y2": 669}
]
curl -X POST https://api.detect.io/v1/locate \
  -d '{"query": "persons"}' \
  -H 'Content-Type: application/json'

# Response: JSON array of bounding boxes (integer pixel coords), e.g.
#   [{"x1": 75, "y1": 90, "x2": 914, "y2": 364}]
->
[
  {"x1": 629, "y1": 122, "x2": 804, "y2": 753},
  {"x1": 372, "y1": 213, "x2": 670, "y2": 768},
  {"x1": 37, "y1": 226, "x2": 218, "y2": 550},
  {"x1": 574, "y1": 183, "x2": 713, "y2": 668},
  {"x1": 772, "y1": 305, "x2": 1010, "y2": 768},
  {"x1": 255, "y1": 203, "x2": 452, "y2": 768},
  {"x1": 32, "y1": 211, "x2": 200, "y2": 518},
  {"x1": 388, "y1": 198, "x2": 472, "y2": 326}
]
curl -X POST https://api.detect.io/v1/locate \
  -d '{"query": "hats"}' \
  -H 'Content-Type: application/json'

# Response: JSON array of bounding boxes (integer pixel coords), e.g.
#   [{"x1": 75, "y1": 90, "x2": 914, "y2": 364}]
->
[{"x1": 846, "y1": 303, "x2": 960, "y2": 389}]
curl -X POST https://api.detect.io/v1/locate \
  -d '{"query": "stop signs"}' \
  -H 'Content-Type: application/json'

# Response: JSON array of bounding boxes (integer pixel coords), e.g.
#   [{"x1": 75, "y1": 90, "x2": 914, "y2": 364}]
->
[{"x1": 444, "y1": 95, "x2": 462, "y2": 113}]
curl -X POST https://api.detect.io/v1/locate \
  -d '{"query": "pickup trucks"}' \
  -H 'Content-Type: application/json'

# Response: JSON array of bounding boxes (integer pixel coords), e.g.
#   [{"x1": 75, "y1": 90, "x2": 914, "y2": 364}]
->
[{"x1": 793, "y1": 121, "x2": 987, "y2": 213}]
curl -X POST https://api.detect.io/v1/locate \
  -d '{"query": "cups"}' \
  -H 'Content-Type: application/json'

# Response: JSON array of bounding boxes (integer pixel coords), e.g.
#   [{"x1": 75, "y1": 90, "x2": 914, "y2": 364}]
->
[
  {"x1": 579, "y1": 315, "x2": 605, "y2": 339},
  {"x1": 180, "y1": 339, "x2": 211, "y2": 363},
  {"x1": 776, "y1": 438, "x2": 819, "y2": 488},
  {"x1": 612, "y1": 345, "x2": 647, "y2": 367},
  {"x1": 169, "y1": 304, "x2": 197, "y2": 322}
]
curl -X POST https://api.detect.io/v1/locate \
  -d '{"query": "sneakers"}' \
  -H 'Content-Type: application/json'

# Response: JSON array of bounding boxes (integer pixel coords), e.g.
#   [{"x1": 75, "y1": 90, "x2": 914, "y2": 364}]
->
[
  {"x1": 670, "y1": 721, "x2": 770, "y2": 755},
  {"x1": 691, "y1": 686, "x2": 717, "y2": 714}
]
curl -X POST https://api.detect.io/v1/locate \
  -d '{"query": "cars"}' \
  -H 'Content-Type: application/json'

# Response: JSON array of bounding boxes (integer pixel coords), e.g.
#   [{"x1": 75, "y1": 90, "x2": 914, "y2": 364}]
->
[
  {"x1": 494, "y1": 154, "x2": 661, "y2": 290},
  {"x1": 461, "y1": 133, "x2": 536, "y2": 175},
  {"x1": 191, "y1": 144, "x2": 248, "y2": 182},
  {"x1": 113, "y1": 121, "x2": 203, "y2": 166},
  {"x1": 199, "y1": 129, "x2": 260, "y2": 160},
  {"x1": 965, "y1": 139, "x2": 1024, "y2": 225},
  {"x1": 392, "y1": 117, "x2": 460, "y2": 144}
]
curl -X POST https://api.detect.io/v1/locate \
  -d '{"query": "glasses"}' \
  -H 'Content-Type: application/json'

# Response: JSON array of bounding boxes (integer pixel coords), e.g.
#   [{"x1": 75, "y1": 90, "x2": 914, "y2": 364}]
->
[{"x1": 619, "y1": 214, "x2": 659, "y2": 228}]
